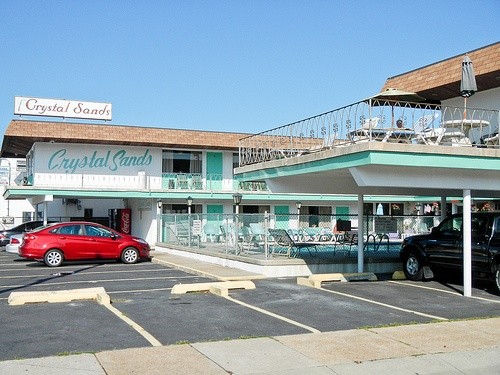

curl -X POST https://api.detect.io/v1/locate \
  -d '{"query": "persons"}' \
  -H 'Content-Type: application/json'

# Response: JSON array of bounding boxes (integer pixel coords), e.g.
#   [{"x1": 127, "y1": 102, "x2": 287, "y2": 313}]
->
[{"x1": 390, "y1": 120, "x2": 412, "y2": 143}]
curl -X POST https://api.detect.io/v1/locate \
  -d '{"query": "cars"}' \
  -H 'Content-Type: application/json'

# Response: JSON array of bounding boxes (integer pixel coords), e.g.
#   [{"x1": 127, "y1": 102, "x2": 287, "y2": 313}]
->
[
  {"x1": 5, "y1": 221, "x2": 64, "y2": 254},
  {"x1": 0, "y1": 221, "x2": 59, "y2": 247},
  {"x1": 18, "y1": 221, "x2": 151, "y2": 267}
]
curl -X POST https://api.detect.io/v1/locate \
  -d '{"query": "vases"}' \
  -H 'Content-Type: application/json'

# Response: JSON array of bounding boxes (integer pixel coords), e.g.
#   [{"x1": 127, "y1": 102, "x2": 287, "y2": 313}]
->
[{"x1": 394, "y1": 210, "x2": 400, "y2": 216}]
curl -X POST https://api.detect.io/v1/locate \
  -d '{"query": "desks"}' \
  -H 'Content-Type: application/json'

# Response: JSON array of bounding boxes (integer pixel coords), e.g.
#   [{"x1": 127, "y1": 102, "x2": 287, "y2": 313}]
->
[{"x1": 439, "y1": 120, "x2": 490, "y2": 147}]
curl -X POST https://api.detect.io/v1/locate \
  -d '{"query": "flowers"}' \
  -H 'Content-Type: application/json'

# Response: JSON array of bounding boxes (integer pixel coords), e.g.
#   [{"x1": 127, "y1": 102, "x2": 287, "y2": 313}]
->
[{"x1": 392, "y1": 203, "x2": 400, "y2": 210}]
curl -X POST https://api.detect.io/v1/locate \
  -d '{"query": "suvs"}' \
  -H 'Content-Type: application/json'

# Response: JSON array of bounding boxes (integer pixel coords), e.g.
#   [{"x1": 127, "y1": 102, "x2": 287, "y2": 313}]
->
[{"x1": 399, "y1": 213, "x2": 500, "y2": 297}]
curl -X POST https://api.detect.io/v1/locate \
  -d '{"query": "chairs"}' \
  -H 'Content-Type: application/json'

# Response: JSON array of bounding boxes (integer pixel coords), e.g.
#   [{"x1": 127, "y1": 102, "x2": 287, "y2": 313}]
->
[
  {"x1": 348, "y1": 112, "x2": 439, "y2": 143},
  {"x1": 267, "y1": 133, "x2": 339, "y2": 159},
  {"x1": 323, "y1": 114, "x2": 380, "y2": 151},
  {"x1": 165, "y1": 220, "x2": 363, "y2": 259}
]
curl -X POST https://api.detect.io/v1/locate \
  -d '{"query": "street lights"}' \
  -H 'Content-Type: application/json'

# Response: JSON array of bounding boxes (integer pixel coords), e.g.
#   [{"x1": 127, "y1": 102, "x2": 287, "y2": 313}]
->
[
  {"x1": 186, "y1": 196, "x2": 194, "y2": 247},
  {"x1": 295, "y1": 201, "x2": 302, "y2": 251},
  {"x1": 232, "y1": 192, "x2": 243, "y2": 255}
]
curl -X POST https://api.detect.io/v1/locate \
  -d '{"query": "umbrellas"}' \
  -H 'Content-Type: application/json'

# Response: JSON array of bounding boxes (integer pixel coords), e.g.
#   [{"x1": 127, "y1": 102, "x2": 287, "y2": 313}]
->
[{"x1": 459, "y1": 56, "x2": 477, "y2": 98}]
